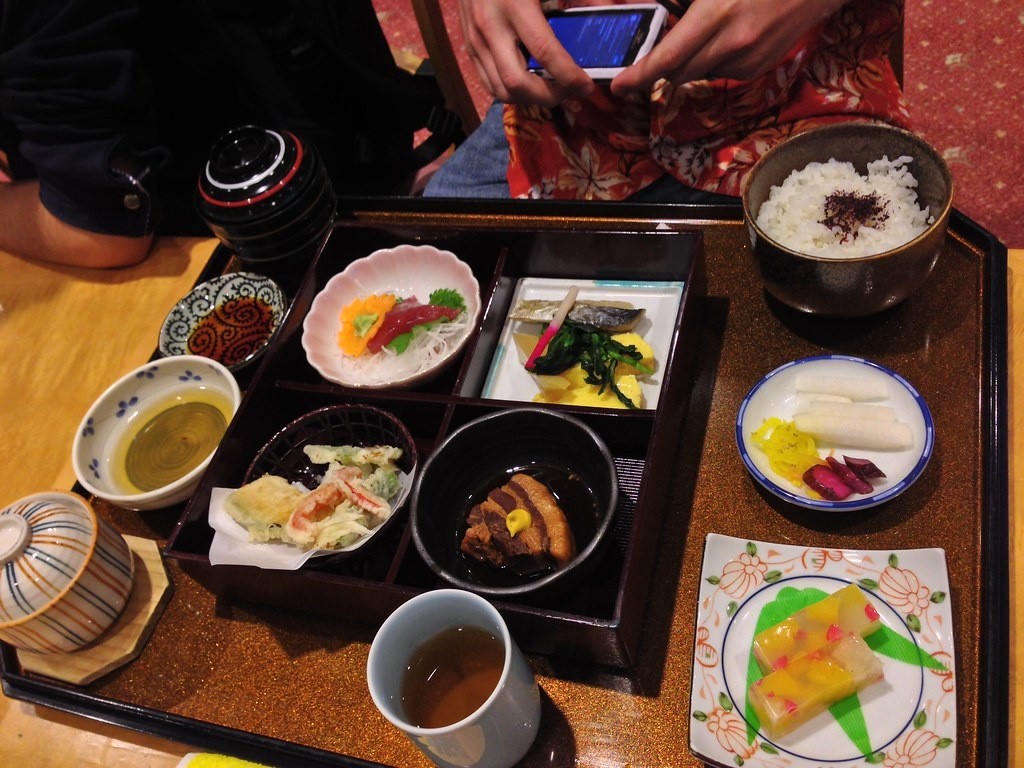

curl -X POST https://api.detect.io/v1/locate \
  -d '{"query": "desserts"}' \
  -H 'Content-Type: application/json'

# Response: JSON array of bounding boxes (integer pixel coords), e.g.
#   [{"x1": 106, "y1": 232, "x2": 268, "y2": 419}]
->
[
  {"x1": 753, "y1": 582, "x2": 880, "y2": 675},
  {"x1": 750, "y1": 633, "x2": 885, "y2": 737}
]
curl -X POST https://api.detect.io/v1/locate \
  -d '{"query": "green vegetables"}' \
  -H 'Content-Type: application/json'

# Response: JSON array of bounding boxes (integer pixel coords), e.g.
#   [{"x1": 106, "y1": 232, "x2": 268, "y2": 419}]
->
[{"x1": 535, "y1": 320, "x2": 653, "y2": 409}]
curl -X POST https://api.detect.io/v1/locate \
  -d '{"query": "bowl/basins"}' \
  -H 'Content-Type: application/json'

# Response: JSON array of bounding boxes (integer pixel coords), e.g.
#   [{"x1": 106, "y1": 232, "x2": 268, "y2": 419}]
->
[
  {"x1": 71, "y1": 355, "x2": 242, "y2": 511},
  {"x1": 157, "y1": 271, "x2": 287, "y2": 373},
  {"x1": 195, "y1": 122, "x2": 338, "y2": 271},
  {"x1": 0, "y1": 489, "x2": 135, "y2": 655},
  {"x1": 301, "y1": 244, "x2": 482, "y2": 391},
  {"x1": 742, "y1": 121, "x2": 954, "y2": 318},
  {"x1": 240, "y1": 403, "x2": 418, "y2": 567},
  {"x1": 410, "y1": 407, "x2": 618, "y2": 595}
]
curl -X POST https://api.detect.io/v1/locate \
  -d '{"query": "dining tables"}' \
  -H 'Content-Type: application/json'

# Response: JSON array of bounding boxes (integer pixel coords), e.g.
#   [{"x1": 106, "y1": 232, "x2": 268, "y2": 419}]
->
[{"x1": 0, "y1": 234, "x2": 1024, "y2": 768}]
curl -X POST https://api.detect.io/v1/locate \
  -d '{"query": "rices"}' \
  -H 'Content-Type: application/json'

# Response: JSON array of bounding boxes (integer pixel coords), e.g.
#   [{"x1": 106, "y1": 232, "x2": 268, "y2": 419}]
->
[{"x1": 755, "y1": 155, "x2": 936, "y2": 258}]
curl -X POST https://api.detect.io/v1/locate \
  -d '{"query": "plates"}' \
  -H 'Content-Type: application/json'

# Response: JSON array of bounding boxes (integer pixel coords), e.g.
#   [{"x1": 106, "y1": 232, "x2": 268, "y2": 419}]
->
[
  {"x1": 480, "y1": 277, "x2": 685, "y2": 411},
  {"x1": 736, "y1": 354, "x2": 935, "y2": 512},
  {"x1": 689, "y1": 533, "x2": 957, "y2": 768}
]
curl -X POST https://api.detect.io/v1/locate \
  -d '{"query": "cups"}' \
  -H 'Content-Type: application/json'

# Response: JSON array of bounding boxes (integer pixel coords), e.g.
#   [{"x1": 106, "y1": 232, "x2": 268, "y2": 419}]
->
[{"x1": 366, "y1": 589, "x2": 541, "y2": 768}]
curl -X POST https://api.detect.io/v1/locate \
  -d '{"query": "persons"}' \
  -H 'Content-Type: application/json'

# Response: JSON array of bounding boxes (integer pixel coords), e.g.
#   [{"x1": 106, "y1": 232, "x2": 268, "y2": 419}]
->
[
  {"x1": 424, "y1": 0, "x2": 913, "y2": 201},
  {"x1": 0, "y1": 0, "x2": 422, "y2": 268}
]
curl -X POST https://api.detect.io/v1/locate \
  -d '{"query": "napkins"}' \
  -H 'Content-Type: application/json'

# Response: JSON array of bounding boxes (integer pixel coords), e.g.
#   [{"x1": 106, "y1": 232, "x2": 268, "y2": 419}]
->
[{"x1": 208, "y1": 467, "x2": 418, "y2": 571}]
